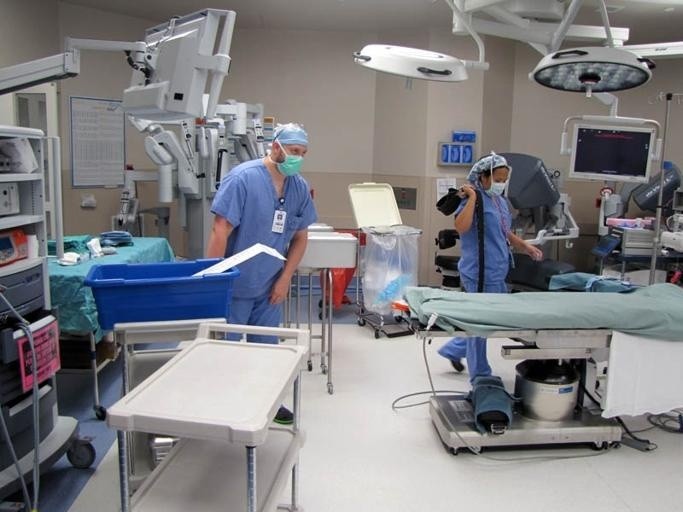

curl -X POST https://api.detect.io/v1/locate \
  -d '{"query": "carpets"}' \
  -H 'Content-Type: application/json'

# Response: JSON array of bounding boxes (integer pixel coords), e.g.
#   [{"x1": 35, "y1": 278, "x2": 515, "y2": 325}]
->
[{"x1": 0, "y1": 340, "x2": 182, "y2": 512}]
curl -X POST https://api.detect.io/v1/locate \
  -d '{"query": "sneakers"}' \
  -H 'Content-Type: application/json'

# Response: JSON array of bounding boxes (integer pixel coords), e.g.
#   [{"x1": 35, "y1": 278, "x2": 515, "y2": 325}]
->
[
  {"x1": 452, "y1": 359, "x2": 465, "y2": 372},
  {"x1": 273, "y1": 406, "x2": 293, "y2": 424}
]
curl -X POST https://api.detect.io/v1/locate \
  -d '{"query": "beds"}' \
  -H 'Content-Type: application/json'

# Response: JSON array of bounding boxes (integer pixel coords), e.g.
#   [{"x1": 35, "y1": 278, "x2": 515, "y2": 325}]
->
[{"x1": 394, "y1": 282, "x2": 683, "y2": 455}]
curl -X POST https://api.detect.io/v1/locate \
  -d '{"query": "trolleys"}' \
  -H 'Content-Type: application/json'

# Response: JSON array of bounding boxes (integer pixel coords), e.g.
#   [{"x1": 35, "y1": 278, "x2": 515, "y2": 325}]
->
[
  {"x1": 349, "y1": 225, "x2": 422, "y2": 339},
  {"x1": 104, "y1": 221, "x2": 357, "y2": 511}
]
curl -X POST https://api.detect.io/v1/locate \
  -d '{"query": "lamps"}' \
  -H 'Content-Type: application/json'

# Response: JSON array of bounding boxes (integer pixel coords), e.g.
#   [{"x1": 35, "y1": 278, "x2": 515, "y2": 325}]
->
[
  {"x1": 352, "y1": 0, "x2": 490, "y2": 89},
  {"x1": 452, "y1": 0, "x2": 683, "y2": 101}
]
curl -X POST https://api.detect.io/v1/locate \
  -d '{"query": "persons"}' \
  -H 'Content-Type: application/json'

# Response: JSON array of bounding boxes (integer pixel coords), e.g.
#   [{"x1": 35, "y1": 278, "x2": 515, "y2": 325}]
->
[
  {"x1": 206, "y1": 120, "x2": 318, "y2": 426},
  {"x1": 437, "y1": 150, "x2": 544, "y2": 387}
]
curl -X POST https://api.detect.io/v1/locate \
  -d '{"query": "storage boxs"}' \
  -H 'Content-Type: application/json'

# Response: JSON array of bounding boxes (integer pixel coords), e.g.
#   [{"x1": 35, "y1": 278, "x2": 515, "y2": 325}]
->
[{"x1": 83, "y1": 257, "x2": 241, "y2": 331}]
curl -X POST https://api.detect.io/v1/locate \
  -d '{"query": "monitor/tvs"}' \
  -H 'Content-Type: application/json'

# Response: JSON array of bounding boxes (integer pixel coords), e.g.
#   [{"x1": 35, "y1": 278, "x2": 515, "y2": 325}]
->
[
  {"x1": 569, "y1": 124, "x2": 656, "y2": 184},
  {"x1": 125, "y1": 16, "x2": 206, "y2": 121}
]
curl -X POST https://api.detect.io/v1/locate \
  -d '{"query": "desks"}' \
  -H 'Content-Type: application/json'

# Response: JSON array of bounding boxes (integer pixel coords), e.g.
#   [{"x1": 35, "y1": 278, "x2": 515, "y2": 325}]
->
[
  {"x1": 599, "y1": 250, "x2": 683, "y2": 283},
  {"x1": 47, "y1": 236, "x2": 178, "y2": 419}
]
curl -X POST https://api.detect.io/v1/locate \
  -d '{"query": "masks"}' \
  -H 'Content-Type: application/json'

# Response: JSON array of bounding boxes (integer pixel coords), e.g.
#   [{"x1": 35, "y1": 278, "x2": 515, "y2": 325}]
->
[
  {"x1": 485, "y1": 169, "x2": 506, "y2": 197},
  {"x1": 272, "y1": 139, "x2": 304, "y2": 176}
]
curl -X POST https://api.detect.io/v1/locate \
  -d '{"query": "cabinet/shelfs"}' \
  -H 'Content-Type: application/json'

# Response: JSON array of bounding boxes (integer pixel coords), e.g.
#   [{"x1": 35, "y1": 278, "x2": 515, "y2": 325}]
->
[{"x1": 0, "y1": 124, "x2": 96, "y2": 501}]
